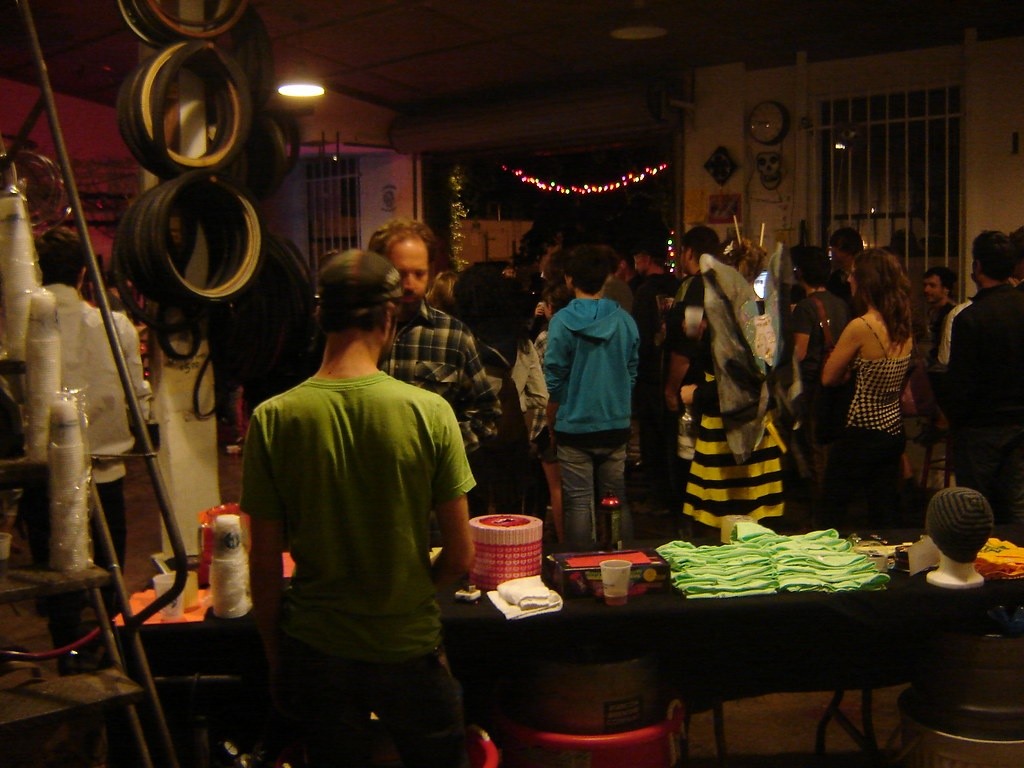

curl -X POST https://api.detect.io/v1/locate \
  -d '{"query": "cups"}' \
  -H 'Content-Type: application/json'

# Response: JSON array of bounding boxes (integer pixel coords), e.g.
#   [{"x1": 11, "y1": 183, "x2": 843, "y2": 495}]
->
[
  {"x1": 0, "y1": 198, "x2": 35, "y2": 360},
  {"x1": 599, "y1": 560, "x2": 632, "y2": 606},
  {"x1": 27, "y1": 290, "x2": 61, "y2": 464},
  {"x1": 895, "y1": 545, "x2": 912, "y2": 571},
  {"x1": 49, "y1": 401, "x2": 88, "y2": 571},
  {"x1": 210, "y1": 514, "x2": 251, "y2": 618},
  {"x1": 0, "y1": 533, "x2": 12, "y2": 559},
  {"x1": 153, "y1": 571, "x2": 198, "y2": 620},
  {"x1": 537, "y1": 302, "x2": 545, "y2": 315}
]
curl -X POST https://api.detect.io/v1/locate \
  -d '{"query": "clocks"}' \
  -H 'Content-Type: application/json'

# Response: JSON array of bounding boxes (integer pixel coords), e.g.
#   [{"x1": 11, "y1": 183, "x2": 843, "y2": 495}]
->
[{"x1": 743, "y1": 97, "x2": 790, "y2": 145}]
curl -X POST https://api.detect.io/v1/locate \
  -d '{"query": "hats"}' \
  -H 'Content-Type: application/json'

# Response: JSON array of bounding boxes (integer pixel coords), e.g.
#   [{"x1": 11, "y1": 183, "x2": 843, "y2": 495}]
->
[
  {"x1": 318, "y1": 244, "x2": 402, "y2": 299},
  {"x1": 927, "y1": 486, "x2": 995, "y2": 563}
]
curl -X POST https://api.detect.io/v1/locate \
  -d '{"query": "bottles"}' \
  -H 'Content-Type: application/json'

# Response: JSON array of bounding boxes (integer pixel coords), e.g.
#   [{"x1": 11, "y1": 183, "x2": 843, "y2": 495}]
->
[
  {"x1": 601, "y1": 490, "x2": 622, "y2": 548},
  {"x1": 678, "y1": 405, "x2": 695, "y2": 460}
]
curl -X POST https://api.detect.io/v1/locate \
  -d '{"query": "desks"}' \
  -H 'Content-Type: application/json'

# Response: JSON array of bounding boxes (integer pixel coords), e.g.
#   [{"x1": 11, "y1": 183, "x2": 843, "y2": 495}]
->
[{"x1": 102, "y1": 526, "x2": 1024, "y2": 768}]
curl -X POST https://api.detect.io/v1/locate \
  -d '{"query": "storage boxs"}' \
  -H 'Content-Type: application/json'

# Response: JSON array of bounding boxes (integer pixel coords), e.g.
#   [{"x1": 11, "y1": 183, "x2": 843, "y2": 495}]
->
[{"x1": 546, "y1": 545, "x2": 671, "y2": 602}]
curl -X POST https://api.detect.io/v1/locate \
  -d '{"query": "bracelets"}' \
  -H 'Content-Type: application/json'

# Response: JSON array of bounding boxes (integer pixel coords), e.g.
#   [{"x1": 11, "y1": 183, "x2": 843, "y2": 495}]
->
[
  {"x1": 667, "y1": 383, "x2": 680, "y2": 391},
  {"x1": 548, "y1": 424, "x2": 554, "y2": 430}
]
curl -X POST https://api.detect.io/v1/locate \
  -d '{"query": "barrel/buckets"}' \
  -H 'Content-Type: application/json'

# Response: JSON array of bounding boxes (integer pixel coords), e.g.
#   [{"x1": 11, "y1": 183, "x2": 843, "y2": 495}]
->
[
  {"x1": 488, "y1": 651, "x2": 683, "y2": 768},
  {"x1": 912, "y1": 631, "x2": 1024, "y2": 739},
  {"x1": 885, "y1": 686, "x2": 1024, "y2": 768},
  {"x1": 519, "y1": 652, "x2": 659, "y2": 733}
]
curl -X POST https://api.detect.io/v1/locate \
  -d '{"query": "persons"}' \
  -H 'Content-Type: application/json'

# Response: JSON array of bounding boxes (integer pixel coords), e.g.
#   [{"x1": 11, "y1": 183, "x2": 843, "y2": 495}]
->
[
  {"x1": 926, "y1": 487, "x2": 994, "y2": 589},
  {"x1": 15, "y1": 224, "x2": 154, "y2": 676},
  {"x1": 237, "y1": 250, "x2": 478, "y2": 768},
  {"x1": 228, "y1": 220, "x2": 1024, "y2": 552}
]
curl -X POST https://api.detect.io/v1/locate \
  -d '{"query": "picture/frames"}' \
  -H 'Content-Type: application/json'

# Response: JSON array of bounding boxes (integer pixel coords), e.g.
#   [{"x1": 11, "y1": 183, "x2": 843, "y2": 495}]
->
[{"x1": 707, "y1": 194, "x2": 742, "y2": 224}]
